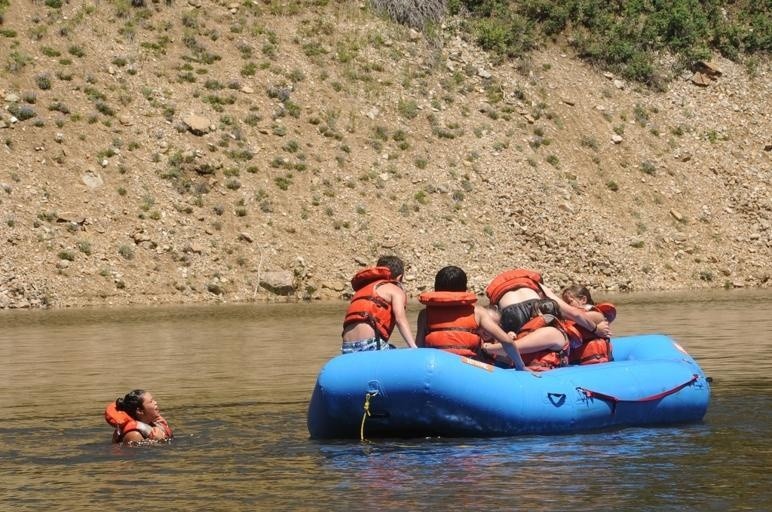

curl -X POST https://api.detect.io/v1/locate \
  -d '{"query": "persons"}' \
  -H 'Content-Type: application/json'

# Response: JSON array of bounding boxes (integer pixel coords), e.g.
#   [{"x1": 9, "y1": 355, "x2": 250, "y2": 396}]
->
[
  {"x1": 112, "y1": 389, "x2": 174, "y2": 448},
  {"x1": 340, "y1": 255, "x2": 418, "y2": 354},
  {"x1": 415, "y1": 265, "x2": 615, "y2": 372}
]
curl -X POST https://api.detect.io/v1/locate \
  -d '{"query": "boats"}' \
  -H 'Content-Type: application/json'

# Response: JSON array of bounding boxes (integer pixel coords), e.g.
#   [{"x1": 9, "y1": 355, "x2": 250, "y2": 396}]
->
[{"x1": 305, "y1": 333, "x2": 713, "y2": 442}]
